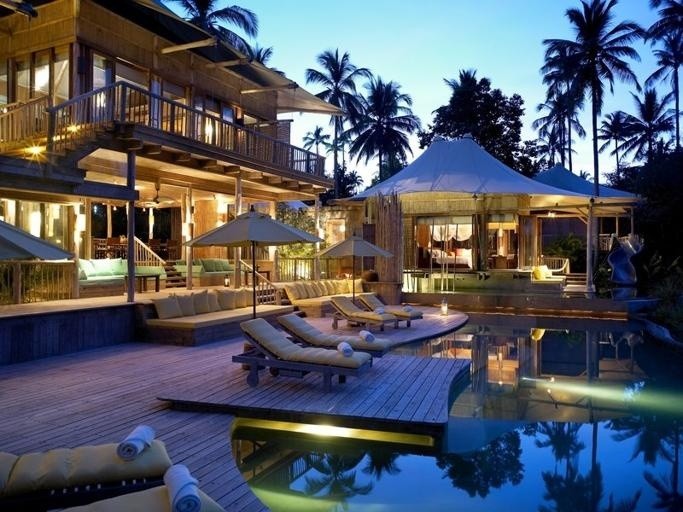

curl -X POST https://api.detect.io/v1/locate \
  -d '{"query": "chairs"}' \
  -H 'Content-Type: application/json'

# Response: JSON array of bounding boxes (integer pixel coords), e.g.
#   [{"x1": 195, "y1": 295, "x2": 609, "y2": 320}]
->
[
  {"x1": 235, "y1": 319, "x2": 374, "y2": 392},
  {"x1": 90, "y1": 235, "x2": 176, "y2": 259},
  {"x1": 328, "y1": 294, "x2": 397, "y2": 334},
  {"x1": 276, "y1": 312, "x2": 391, "y2": 370},
  {"x1": 0, "y1": 441, "x2": 170, "y2": 501},
  {"x1": 53, "y1": 481, "x2": 226, "y2": 512},
  {"x1": 356, "y1": 292, "x2": 425, "y2": 330}
]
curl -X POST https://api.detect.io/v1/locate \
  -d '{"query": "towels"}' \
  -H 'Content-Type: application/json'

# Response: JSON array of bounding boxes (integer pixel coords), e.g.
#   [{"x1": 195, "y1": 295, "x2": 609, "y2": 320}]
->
[
  {"x1": 162, "y1": 463, "x2": 201, "y2": 512},
  {"x1": 374, "y1": 309, "x2": 384, "y2": 314},
  {"x1": 401, "y1": 305, "x2": 413, "y2": 312},
  {"x1": 337, "y1": 341, "x2": 354, "y2": 356},
  {"x1": 358, "y1": 329, "x2": 375, "y2": 343},
  {"x1": 116, "y1": 425, "x2": 153, "y2": 459}
]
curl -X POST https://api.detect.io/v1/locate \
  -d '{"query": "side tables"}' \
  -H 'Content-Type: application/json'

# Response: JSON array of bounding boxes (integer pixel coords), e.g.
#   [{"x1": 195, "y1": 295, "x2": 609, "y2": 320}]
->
[
  {"x1": 126, "y1": 273, "x2": 160, "y2": 293},
  {"x1": 240, "y1": 268, "x2": 272, "y2": 287}
]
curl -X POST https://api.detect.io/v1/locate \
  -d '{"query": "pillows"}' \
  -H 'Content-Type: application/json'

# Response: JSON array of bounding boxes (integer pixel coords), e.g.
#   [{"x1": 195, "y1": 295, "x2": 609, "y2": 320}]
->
[
  {"x1": 539, "y1": 264, "x2": 552, "y2": 277},
  {"x1": 455, "y1": 247, "x2": 461, "y2": 255},
  {"x1": 176, "y1": 256, "x2": 229, "y2": 272},
  {"x1": 534, "y1": 268, "x2": 545, "y2": 280},
  {"x1": 285, "y1": 278, "x2": 365, "y2": 299},
  {"x1": 460, "y1": 248, "x2": 465, "y2": 256},
  {"x1": 151, "y1": 288, "x2": 254, "y2": 320},
  {"x1": 76, "y1": 257, "x2": 125, "y2": 280}
]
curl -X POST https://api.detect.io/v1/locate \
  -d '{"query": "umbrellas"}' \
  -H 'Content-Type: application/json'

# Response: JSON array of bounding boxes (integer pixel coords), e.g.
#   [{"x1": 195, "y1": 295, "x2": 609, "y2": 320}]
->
[
  {"x1": 312, "y1": 230, "x2": 394, "y2": 305},
  {"x1": 180, "y1": 204, "x2": 326, "y2": 320}
]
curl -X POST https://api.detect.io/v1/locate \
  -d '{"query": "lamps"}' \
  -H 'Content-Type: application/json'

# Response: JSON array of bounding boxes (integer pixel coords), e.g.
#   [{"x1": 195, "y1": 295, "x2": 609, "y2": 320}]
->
[
  {"x1": 440, "y1": 295, "x2": 449, "y2": 317},
  {"x1": 223, "y1": 274, "x2": 230, "y2": 286}
]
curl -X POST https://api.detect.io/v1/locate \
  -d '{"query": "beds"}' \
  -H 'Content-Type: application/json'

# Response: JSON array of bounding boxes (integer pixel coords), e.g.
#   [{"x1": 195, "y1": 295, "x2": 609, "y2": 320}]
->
[{"x1": 421, "y1": 246, "x2": 471, "y2": 268}]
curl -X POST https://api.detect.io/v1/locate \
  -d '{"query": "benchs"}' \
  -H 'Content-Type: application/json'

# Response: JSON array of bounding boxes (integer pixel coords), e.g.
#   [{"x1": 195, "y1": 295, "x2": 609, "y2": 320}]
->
[
  {"x1": 44, "y1": 258, "x2": 167, "y2": 298},
  {"x1": 286, "y1": 285, "x2": 377, "y2": 316},
  {"x1": 135, "y1": 302, "x2": 296, "y2": 346},
  {"x1": 173, "y1": 260, "x2": 254, "y2": 287}
]
